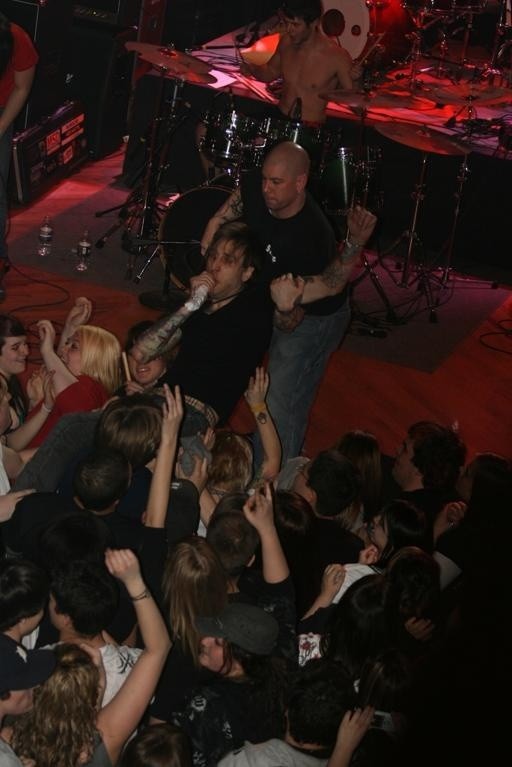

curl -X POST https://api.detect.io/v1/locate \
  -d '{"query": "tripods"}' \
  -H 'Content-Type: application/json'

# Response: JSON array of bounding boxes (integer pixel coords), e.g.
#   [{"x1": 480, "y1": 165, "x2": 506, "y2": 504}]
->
[
  {"x1": 351, "y1": 153, "x2": 448, "y2": 323},
  {"x1": 395, "y1": 122, "x2": 501, "y2": 323},
  {"x1": 96, "y1": 71, "x2": 166, "y2": 249}
]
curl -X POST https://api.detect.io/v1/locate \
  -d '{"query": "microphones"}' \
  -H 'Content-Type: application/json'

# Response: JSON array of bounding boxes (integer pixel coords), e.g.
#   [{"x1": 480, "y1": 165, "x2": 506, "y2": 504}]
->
[{"x1": 184, "y1": 275, "x2": 214, "y2": 312}]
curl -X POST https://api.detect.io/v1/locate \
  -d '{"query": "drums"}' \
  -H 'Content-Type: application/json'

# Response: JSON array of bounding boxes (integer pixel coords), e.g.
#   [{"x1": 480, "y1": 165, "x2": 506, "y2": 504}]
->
[
  {"x1": 157, "y1": 172, "x2": 259, "y2": 295},
  {"x1": 319, "y1": 1, "x2": 411, "y2": 73},
  {"x1": 199, "y1": 107, "x2": 300, "y2": 167}
]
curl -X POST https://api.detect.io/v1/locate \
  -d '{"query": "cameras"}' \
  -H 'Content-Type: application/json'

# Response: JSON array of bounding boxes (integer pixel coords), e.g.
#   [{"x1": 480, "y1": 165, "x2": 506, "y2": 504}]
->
[{"x1": 367, "y1": 711, "x2": 396, "y2": 731}]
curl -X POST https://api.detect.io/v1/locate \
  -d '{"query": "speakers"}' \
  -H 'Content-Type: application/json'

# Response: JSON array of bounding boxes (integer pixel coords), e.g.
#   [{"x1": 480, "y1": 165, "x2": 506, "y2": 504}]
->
[{"x1": 65, "y1": 19, "x2": 137, "y2": 159}]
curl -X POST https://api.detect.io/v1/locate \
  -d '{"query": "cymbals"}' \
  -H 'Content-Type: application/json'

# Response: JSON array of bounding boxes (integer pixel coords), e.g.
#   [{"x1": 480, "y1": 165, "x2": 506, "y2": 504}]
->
[
  {"x1": 153, "y1": 63, "x2": 217, "y2": 83},
  {"x1": 374, "y1": 121, "x2": 472, "y2": 156},
  {"x1": 432, "y1": 84, "x2": 504, "y2": 104},
  {"x1": 318, "y1": 89, "x2": 410, "y2": 109},
  {"x1": 126, "y1": 42, "x2": 213, "y2": 73}
]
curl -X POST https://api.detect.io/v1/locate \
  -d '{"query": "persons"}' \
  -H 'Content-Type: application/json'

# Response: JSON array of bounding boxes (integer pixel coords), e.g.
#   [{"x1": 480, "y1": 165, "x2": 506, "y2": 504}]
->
[
  {"x1": 1, "y1": 294, "x2": 511, "y2": 764},
  {"x1": 1, "y1": 14, "x2": 39, "y2": 275},
  {"x1": 236, "y1": 0, "x2": 363, "y2": 129},
  {"x1": 200, "y1": 141, "x2": 356, "y2": 491},
  {"x1": 4, "y1": 204, "x2": 381, "y2": 498}
]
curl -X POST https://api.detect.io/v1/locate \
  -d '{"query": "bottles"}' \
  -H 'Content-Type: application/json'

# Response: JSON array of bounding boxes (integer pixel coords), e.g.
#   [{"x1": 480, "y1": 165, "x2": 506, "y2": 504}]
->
[
  {"x1": 487, "y1": 72, "x2": 494, "y2": 89},
  {"x1": 75, "y1": 229, "x2": 93, "y2": 271},
  {"x1": 38, "y1": 215, "x2": 55, "y2": 256},
  {"x1": 363, "y1": 59, "x2": 372, "y2": 91}
]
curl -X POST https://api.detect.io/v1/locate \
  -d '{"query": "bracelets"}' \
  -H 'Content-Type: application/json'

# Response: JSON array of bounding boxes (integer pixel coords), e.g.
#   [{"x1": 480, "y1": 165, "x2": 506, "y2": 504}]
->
[
  {"x1": 276, "y1": 305, "x2": 295, "y2": 314},
  {"x1": 343, "y1": 225, "x2": 364, "y2": 250}
]
